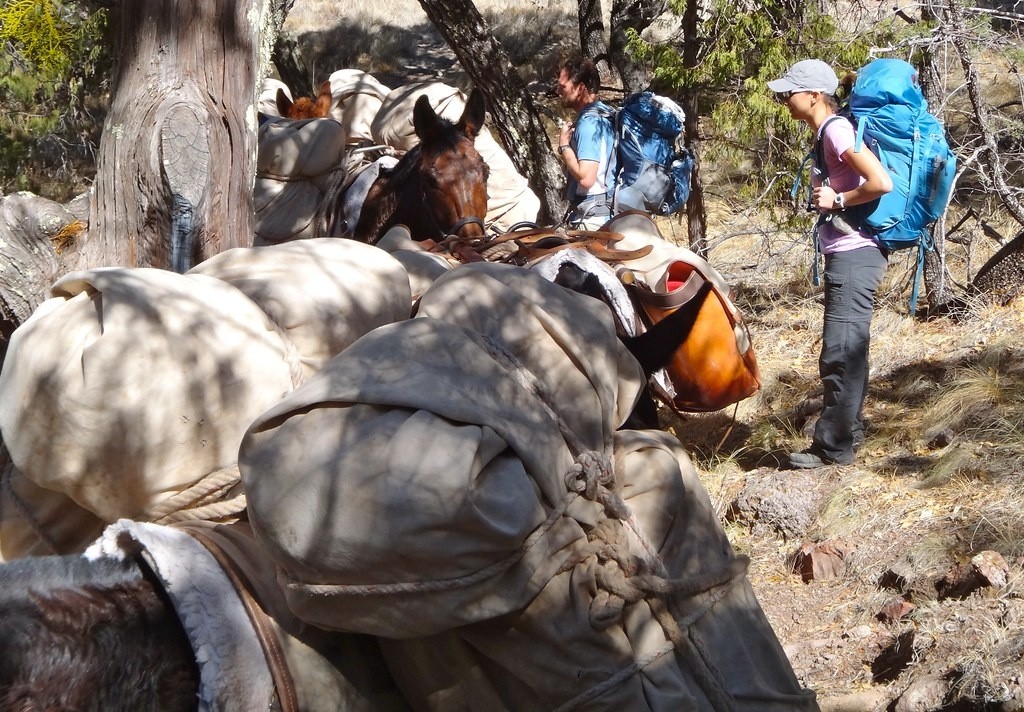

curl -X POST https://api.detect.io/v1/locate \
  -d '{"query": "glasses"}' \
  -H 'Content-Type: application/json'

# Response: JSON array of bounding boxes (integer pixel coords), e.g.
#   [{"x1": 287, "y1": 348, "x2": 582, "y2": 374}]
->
[{"x1": 788, "y1": 88, "x2": 814, "y2": 96}]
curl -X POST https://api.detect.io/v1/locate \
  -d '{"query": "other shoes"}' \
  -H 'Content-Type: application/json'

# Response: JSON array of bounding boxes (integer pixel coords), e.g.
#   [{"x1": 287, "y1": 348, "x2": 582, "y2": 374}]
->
[
  {"x1": 851, "y1": 428, "x2": 864, "y2": 447},
  {"x1": 790, "y1": 449, "x2": 823, "y2": 469}
]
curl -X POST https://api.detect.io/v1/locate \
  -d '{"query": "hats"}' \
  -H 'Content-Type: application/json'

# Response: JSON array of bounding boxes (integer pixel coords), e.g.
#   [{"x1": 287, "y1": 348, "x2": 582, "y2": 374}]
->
[{"x1": 767, "y1": 59, "x2": 838, "y2": 96}]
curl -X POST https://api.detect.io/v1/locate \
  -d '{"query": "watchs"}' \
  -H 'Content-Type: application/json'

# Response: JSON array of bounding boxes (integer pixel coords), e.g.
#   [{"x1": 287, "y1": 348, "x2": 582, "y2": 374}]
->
[
  {"x1": 558, "y1": 145, "x2": 571, "y2": 155},
  {"x1": 835, "y1": 192, "x2": 847, "y2": 211}
]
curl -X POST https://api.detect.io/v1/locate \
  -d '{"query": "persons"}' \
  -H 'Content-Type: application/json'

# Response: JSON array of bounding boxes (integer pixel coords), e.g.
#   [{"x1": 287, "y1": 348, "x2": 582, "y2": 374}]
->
[
  {"x1": 766, "y1": 59, "x2": 893, "y2": 468},
  {"x1": 557, "y1": 58, "x2": 618, "y2": 233}
]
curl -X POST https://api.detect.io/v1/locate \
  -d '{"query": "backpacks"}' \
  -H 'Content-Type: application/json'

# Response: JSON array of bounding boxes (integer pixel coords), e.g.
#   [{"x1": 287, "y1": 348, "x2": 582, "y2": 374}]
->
[
  {"x1": 791, "y1": 59, "x2": 956, "y2": 314},
  {"x1": 563, "y1": 90, "x2": 691, "y2": 234},
  {"x1": 586, "y1": 211, "x2": 763, "y2": 417}
]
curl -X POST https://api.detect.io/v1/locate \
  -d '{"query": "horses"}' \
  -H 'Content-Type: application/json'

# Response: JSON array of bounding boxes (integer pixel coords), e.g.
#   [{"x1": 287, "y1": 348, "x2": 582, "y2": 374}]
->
[
  {"x1": 0, "y1": 555, "x2": 202, "y2": 712},
  {"x1": 275, "y1": 80, "x2": 331, "y2": 121},
  {"x1": 334, "y1": 86, "x2": 490, "y2": 261}
]
